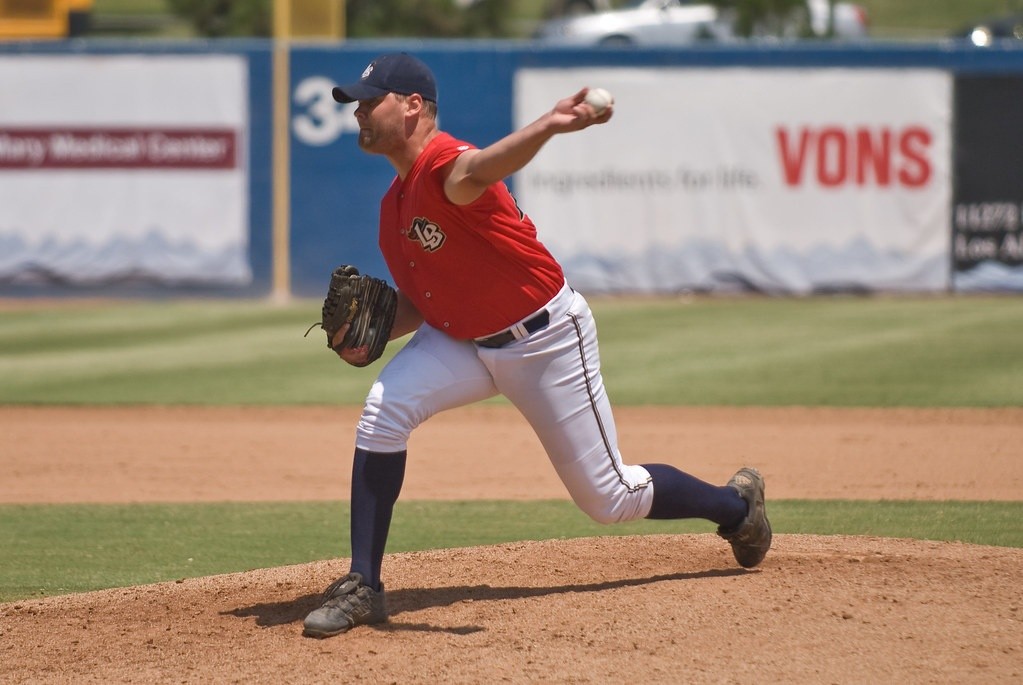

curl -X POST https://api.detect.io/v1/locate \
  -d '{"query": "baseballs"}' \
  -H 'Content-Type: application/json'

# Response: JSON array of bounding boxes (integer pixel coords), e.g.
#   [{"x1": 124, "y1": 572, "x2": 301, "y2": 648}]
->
[{"x1": 585, "y1": 88, "x2": 611, "y2": 112}]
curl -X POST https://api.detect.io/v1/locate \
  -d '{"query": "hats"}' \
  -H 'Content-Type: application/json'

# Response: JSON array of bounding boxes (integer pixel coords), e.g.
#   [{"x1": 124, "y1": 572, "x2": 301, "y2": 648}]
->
[{"x1": 331, "y1": 52, "x2": 440, "y2": 104}]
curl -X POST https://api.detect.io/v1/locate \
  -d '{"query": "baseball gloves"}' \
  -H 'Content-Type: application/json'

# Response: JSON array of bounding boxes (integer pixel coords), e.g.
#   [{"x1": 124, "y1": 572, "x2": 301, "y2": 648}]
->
[{"x1": 304, "y1": 263, "x2": 397, "y2": 368}]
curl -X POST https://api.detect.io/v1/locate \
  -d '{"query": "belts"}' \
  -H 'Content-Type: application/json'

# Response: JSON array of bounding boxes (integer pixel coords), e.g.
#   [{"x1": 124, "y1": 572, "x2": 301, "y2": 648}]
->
[{"x1": 474, "y1": 311, "x2": 551, "y2": 350}]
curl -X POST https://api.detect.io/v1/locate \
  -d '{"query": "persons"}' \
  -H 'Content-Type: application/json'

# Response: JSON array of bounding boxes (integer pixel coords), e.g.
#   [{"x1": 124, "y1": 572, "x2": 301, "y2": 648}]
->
[{"x1": 302, "y1": 50, "x2": 772, "y2": 639}]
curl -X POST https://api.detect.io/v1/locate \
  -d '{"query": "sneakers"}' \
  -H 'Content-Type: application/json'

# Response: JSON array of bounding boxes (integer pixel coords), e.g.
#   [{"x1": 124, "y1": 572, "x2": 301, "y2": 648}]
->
[
  {"x1": 716, "y1": 467, "x2": 773, "y2": 568},
  {"x1": 303, "y1": 572, "x2": 388, "y2": 638}
]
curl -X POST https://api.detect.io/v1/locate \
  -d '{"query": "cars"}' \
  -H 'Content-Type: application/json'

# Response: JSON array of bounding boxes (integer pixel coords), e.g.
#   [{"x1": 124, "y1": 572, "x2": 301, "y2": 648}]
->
[{"x1": 530, "y1": 0, "x2": 868, "y2": 48}]
